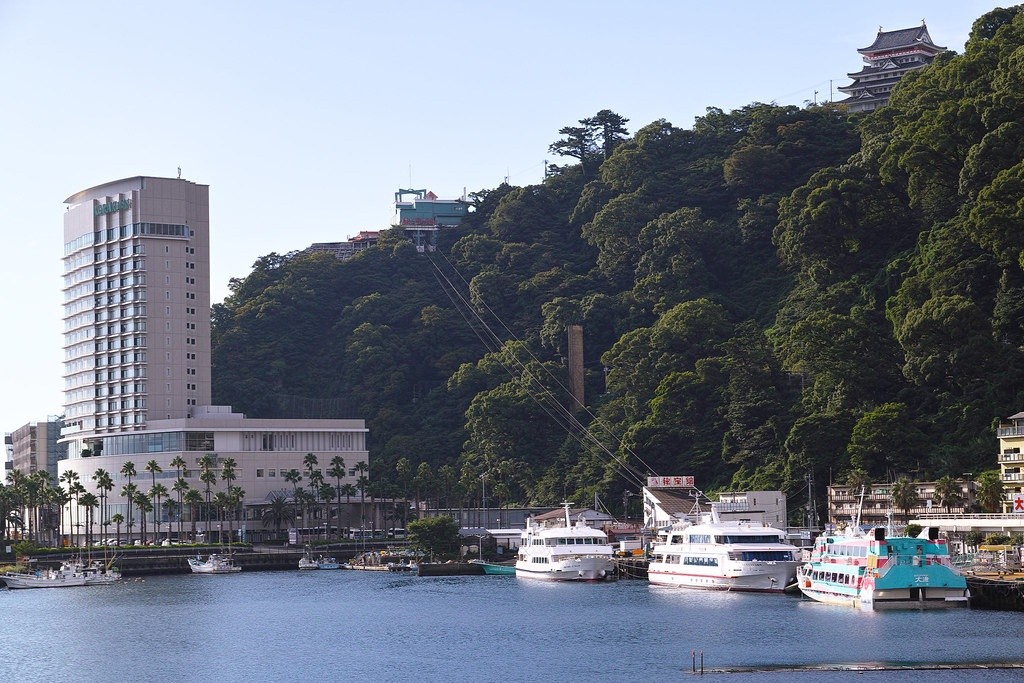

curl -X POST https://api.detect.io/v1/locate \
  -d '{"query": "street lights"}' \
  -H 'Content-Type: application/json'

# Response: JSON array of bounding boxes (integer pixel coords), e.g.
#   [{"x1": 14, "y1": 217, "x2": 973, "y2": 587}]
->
[
  {"x1": 323, "y1": 522, "x2": 327, "y2": 541},
  {"x1": 479, "y1": 472, "x2": 486, "y2": 529},
  {"x1": 369, "y1": 522, "x2": 374, "y2": 539}
]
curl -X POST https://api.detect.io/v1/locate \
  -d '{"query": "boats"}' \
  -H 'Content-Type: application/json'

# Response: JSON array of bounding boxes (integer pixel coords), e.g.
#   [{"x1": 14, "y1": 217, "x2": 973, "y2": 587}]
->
[
  {"x1": 298, "y1": 547, "x2": 516, "y2": 576},
  {"x1": 0, "y1": 547, "x2": 125, "y2": 588},
  {"x1": 187, "y1": 550, "x2": 242, "y2": 574},
  {"x1": 797, "y1": 485, "x2": 970, "y2": 612},
  {"x1": 514, "y1": 500, "x2": 614, "y2": 582},
  {"x1": 646, "y1": 490, "x2": 809, "y2": 591}
]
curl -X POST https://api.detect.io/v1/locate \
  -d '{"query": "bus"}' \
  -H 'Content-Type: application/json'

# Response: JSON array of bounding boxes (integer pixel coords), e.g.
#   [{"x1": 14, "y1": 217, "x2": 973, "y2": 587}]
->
[
  {"x1": 348, "y1": 528, "x2": 406, "y2": 541},
  {"x1": 289, "y1": 525, "x2": 345, "y2": 545}
]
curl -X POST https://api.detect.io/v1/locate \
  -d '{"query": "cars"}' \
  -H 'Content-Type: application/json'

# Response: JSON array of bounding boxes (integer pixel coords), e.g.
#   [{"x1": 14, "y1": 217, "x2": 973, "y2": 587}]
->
[{"x1": 84, "y1": 538, "x2": 190, "y2": 546}]
